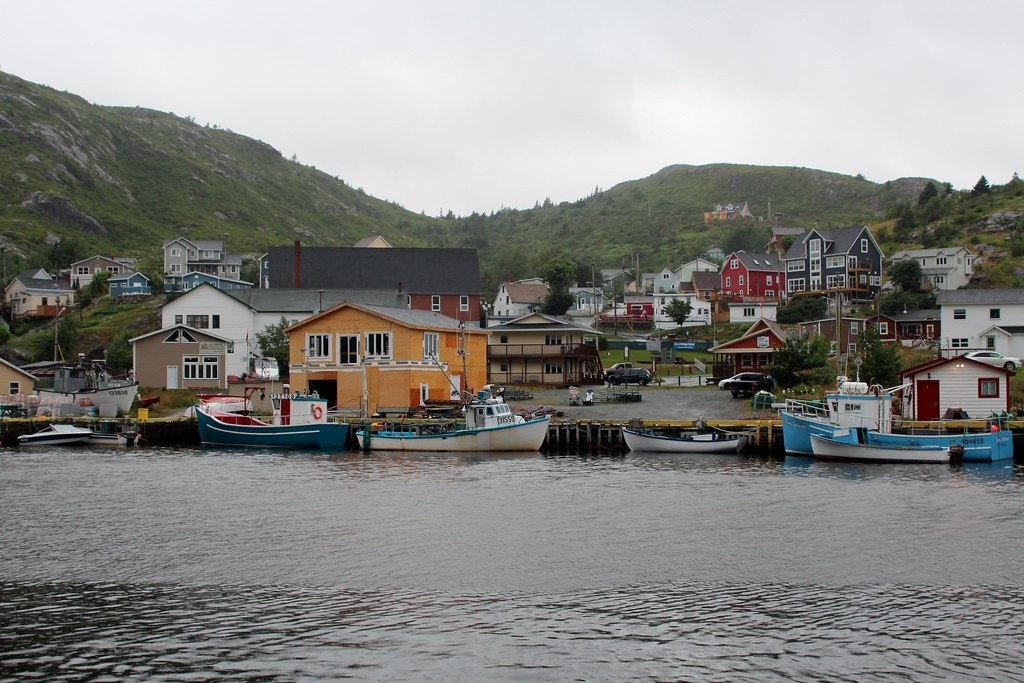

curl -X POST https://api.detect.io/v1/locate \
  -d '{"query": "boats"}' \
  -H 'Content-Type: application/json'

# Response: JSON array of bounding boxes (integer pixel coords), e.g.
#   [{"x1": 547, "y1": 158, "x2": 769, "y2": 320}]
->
[
  {"x1": 354, "y1": 383, "x2": 555, "y2": 453},
  {"x1": 15, "y1": 424, "x2": 92, "y2": 444},
  {"x1": 809, "y1": 426, "x2": 964, "y2": 464},
  {"x1": 185, "y1": 394, "x2": 252, "y2": 417},
  {"x1": 196, "y1": 385, "x2": 349, "y2": 450},
  {"x1": 781, "y1": 353, "x2": 1014, "y2": 462},
  {"x1": 30, "y1": 353, "x2": 138, "y2": 413},
  {"x1": 621, "y1": 420, "x2": 746, "y2": 452},
  {"x1": 84, "y1": 430, "x2": 143, "y2": 446}
]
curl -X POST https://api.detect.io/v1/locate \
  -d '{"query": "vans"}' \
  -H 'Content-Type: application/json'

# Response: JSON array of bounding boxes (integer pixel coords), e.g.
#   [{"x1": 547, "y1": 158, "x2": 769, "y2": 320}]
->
[{"x1": 254, "y1": 357, "x2": 279, "y2": 381}]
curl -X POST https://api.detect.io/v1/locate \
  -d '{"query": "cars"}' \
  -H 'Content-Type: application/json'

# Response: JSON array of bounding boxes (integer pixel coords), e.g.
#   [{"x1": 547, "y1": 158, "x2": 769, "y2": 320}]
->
[
  {"x1": 717, "y1": 372, "x2": 771, "y2": 389},
  {"x1": 963, "y1": 350, "x2": 1022, "y2": 370}
]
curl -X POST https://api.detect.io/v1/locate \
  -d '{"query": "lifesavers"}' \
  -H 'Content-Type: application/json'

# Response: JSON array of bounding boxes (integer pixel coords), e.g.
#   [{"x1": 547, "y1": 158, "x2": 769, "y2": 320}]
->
[{"x1": 314, "y1": 406, "x2": 323, "y2": 419}]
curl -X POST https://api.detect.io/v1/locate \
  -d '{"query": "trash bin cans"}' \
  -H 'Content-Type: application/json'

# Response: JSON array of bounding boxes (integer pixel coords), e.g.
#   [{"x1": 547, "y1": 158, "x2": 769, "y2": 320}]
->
[{"x1": 138, "y1": 408, "x2": 149, "y2": 419}]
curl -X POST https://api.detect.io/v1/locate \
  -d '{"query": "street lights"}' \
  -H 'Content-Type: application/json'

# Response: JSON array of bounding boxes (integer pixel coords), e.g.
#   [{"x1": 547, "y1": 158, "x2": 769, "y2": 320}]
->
[
  {"x1": 586, "y1": 282, "x2": 596, "y2": 328},
  {"x1": 54, "y1": 307, "x2": 66, "y2": 361},
  {"x1": 705, "y1": 311, "x2": 716, "y2": 348},
  {"x1": 318, "y1": 290, "x2": 324, "y2": 312},
  {"x1": 224, "y1": 233, "x2": 228, "y2": 278}
]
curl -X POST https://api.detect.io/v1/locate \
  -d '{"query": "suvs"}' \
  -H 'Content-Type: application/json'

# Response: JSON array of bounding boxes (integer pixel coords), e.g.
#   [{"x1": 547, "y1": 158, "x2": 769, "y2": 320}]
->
[{"x1": 608, "y1": 368, "x2": 651, "y2": 386}]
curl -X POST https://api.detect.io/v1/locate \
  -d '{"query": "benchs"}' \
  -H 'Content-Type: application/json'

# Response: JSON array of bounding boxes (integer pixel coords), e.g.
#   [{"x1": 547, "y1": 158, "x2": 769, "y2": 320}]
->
[{"x1": 567, "y1": 390, "x2": 641, "y2": 403}]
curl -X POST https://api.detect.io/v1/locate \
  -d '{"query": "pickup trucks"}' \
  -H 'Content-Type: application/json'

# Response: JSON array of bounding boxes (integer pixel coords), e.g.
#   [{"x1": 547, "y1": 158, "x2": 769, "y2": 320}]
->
[
  {"x1": 604, "y1": 362, "x2": 648, "y2": 382},
  {"x1": 730, "y1": 373, "x2": 772, "y2": 398}
]
